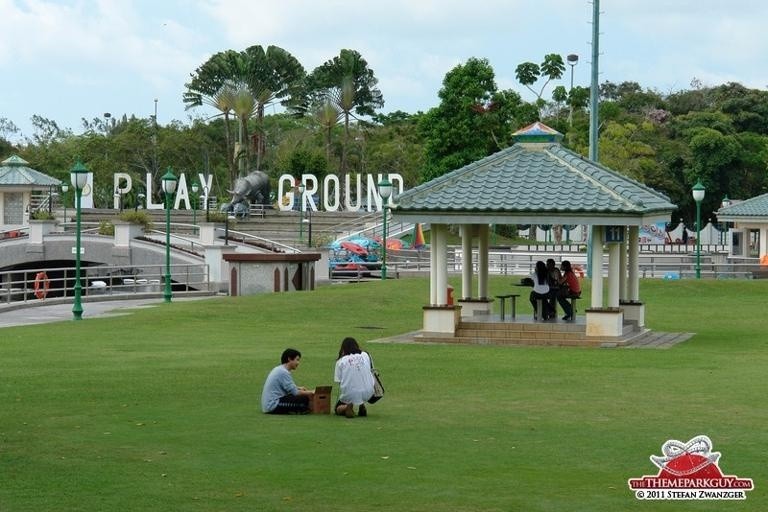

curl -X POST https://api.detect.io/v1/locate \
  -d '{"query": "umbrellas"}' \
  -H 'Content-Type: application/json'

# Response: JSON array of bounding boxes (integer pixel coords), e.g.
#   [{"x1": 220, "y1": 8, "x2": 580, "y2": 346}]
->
[{"x1": 411, "y1": 222, "x2": 425, "y2": 262}]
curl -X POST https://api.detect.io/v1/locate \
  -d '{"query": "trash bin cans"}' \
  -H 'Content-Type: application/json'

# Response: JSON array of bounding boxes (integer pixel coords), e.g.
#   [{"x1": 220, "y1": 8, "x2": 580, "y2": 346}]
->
[{"x1": 447, "y1": 284, "x2": 454, "y2": 305}]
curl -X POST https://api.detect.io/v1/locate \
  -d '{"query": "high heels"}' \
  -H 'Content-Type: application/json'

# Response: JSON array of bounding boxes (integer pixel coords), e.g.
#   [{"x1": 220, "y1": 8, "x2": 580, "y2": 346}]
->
[{"x1": 562, "y1": 311, "x2": 572, "y2": 320}]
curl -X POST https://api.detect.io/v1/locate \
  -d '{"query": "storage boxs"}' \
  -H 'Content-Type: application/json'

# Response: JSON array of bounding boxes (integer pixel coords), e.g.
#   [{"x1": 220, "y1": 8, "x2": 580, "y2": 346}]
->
[{"x1": 306, "y1": 386, "x2": 332, "y2": 414}]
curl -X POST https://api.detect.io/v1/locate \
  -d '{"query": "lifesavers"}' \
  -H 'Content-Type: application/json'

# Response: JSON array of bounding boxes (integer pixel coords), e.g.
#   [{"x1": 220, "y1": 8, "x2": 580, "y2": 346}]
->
[
  {"x1": 35, "y1": 272, "x2": 49, "y2": 299},
  {"x1": 570, "y1": 267, "x2": 584, "y2": 277}
]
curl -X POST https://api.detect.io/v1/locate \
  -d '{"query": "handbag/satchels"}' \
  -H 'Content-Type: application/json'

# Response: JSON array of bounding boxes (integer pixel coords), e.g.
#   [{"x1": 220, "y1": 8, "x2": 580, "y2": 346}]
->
[{"x1": 364, "y1": 351, "x2": 384, "y2": 403}]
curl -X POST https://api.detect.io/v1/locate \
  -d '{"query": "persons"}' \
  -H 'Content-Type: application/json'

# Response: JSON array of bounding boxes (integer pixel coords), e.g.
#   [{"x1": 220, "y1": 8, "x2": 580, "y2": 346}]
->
[
  {"x1": 261, "y1": 348, "x2": 315, "y2": 415},
  {"x1": 528, "y1": 261, "x2": 555, "y2": 320},
  {"x1": 333, "y1": 337, "x2": 375, "y2": 417},
  {"x1": 545, "y1": 259, "x2": 563, "y2": 318},
  {"x1": 682, "y1": 227, "x2": 688, "y2": 244},
  {"x1": 557, "y1": 260, "x2": 581, "y2": 320}
]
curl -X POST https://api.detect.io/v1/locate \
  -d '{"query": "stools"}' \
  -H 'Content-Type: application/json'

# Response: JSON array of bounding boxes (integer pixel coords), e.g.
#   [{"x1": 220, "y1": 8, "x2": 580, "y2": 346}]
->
[
  {"x1": 496, "y1": 296, "x2": 511, "y2": 321},
  {"x1": 535, "y1": 297, "x2": 583, "y2": 321},
  {"x1": 507, "y1": 295, "x2": 520, "y2": 318}
]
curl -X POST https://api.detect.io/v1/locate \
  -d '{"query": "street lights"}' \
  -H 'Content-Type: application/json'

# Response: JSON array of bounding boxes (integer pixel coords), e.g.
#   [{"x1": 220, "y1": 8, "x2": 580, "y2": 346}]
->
[
  {"x1": 297, "y1": 182, "x2": 315, "y2": 252},
  {"x1": 153, "y1": 97, "x2": 158, "y2": 118},
  {"x1": 225, "y1": 208, "x2": 235, "y2": 245},
  {"x1": 160, "y1": 167, "x2": 179, "y2": 305},
  {"x1": 70, "y1": 160, "x2": 90, "y2": 322},
  {"x1": 48, "y1": 182, "x2": 60, "y2": 216},
  {"x1": 112, "y1": 185, "x2": 123, "y2": 215},
  {"x1": 691, "y1": 177, "x2": 706, "y2": 280},
  {"x1": 567, "y1": 54, "x2": 578, "y2": 149},
  {"x1": 191, "y1": 181, "x2": 210, "y2": 238},
  {"x1": 721, "y1": 195, "x2": 731, "y2": 246},
  {"x1": 61, "y1": 182, "x2": 68, "y2": 231},
  {"x1": 104, "y1": 113, "x2": 111, "y2": 210},
  {"x1": 377, "y1": 173, "x2": 393, "y2": 281}
]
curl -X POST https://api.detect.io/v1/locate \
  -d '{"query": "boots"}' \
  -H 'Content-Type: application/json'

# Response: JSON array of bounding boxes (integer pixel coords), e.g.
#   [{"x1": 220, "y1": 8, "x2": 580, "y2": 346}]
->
[{"x1": 336, "y1": 404, "x2": 354, "y2": 417}]
